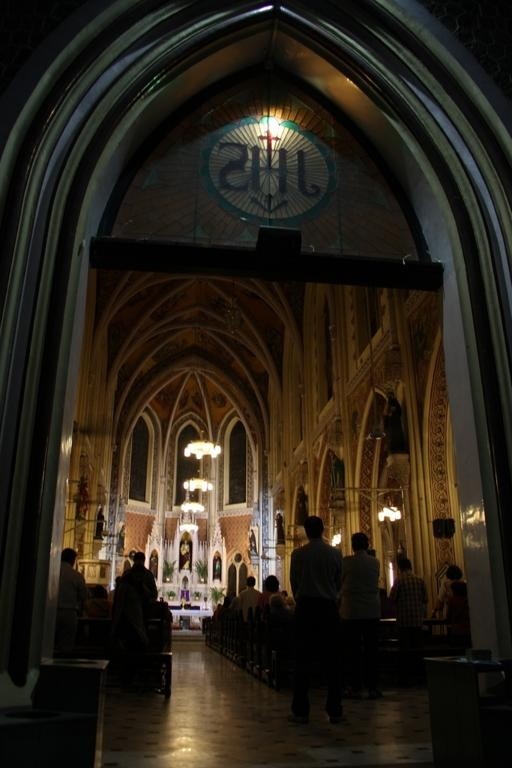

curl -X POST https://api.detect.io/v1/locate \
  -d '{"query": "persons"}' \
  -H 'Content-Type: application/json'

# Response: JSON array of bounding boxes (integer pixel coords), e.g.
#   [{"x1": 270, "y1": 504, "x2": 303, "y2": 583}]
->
[
  {"x1": 388, "y1": 557, "x2": 428, "y2": 687},
  {"x1": 338, "y1": 532, "x2": 383, "y2": 698},
  {"x1": 52, "y1": 546, "x2": 172, "y2": 698},
  {"x1": 380, "y1": 588, "x2": 388, "y2": 600},
  {"x1": 211, "y1": 575, "x2": 296, "y2": 664},
  {"x1": 287, "y1": 514, "x2": 348, "y2": 723},
  {"x1": 446, "y1": 580, "x2": 471, "y2": 634},
  {"x1": 431, "y1": 564, "x2": 465, "y2": 617}
]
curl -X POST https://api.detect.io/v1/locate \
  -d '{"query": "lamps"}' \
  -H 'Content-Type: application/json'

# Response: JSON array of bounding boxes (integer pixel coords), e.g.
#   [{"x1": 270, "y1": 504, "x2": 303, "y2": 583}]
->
[{"x1": 176, "y1": 332, "x2": 224, "y2": 532}]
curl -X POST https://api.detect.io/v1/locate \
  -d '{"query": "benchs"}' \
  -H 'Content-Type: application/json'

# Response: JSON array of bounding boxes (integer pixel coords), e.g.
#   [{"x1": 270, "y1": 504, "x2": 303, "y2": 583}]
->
[
  {"x1": 202, "y1": 607, "x2": 470, "y2": 695},
  {"x1": 75, "y1": 600, "x2": 175, "y2": 700}
]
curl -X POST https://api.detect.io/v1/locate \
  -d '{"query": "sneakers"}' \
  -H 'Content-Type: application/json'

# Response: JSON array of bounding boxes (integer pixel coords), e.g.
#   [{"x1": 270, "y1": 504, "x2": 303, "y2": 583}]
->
[
  {"x1": 287, "y1": 712, "x2": 309, "y2": 723},
  {"x1": 328, "y1": 715, "x2": 348, "y2": 725}
]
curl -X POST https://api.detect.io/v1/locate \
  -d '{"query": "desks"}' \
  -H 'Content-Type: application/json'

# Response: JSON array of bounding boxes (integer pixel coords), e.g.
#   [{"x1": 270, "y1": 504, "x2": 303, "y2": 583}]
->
[{"x1": 168, "y1": 604, "x2": 214, "y2": 632}]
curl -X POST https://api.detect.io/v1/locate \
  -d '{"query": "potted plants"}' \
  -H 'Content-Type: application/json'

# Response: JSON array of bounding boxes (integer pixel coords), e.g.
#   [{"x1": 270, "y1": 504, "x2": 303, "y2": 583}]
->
[
  {"x1": 166, "y1": 590, "x2": 177, "y2": 600},
  {"x1": 193, "y1": 557, "x2": 208, "y2": 584},
  {"x1": 163, "y1": 559, "x2": 177, "y2": 581},
  {"x1": 209, "y1": 585, "x2": 227, "y2": 613},
  {"x1": 191, "y1": 591, "x2": 203, "y2": 601}
]
